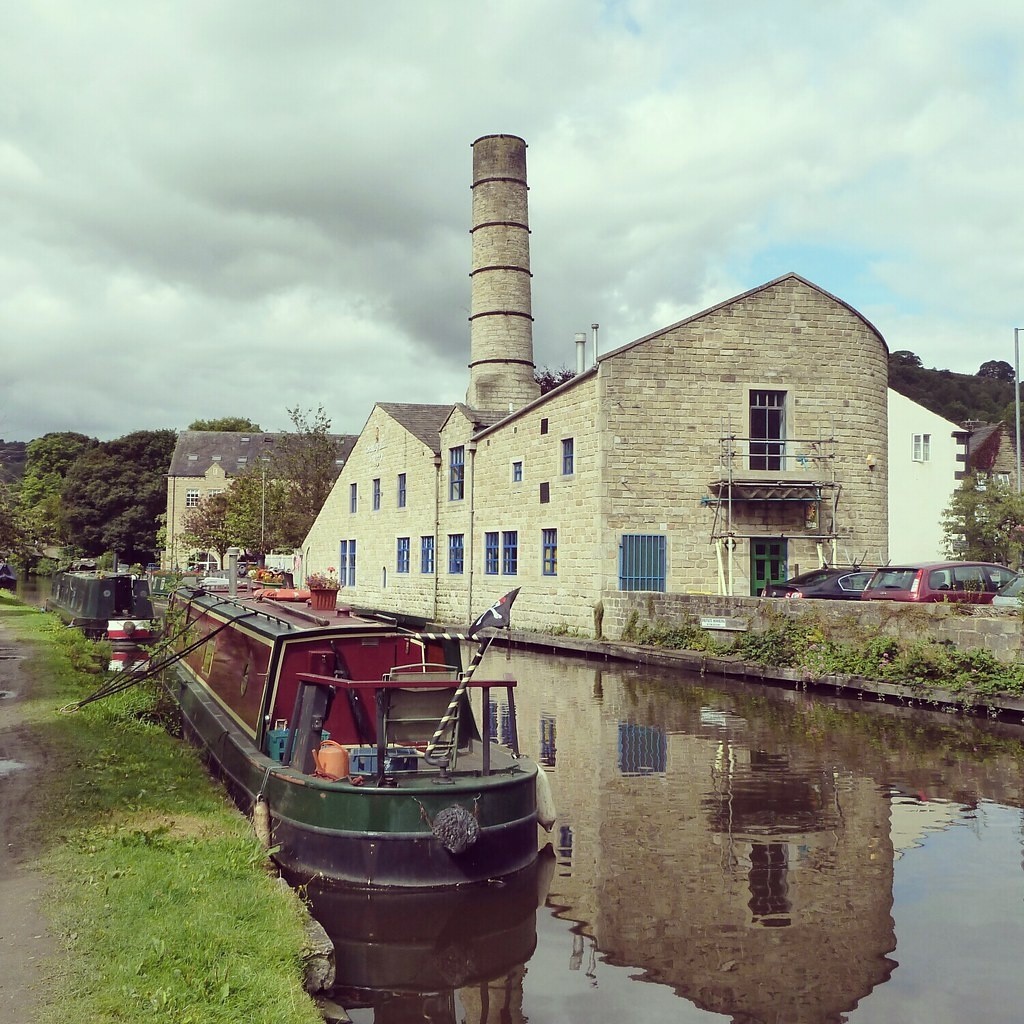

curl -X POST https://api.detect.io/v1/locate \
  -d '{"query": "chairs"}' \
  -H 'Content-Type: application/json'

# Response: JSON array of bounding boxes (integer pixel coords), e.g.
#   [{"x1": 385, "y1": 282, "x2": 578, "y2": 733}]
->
[{"x1": 934, "y1": 573, "x2": 950, "y2": 589}]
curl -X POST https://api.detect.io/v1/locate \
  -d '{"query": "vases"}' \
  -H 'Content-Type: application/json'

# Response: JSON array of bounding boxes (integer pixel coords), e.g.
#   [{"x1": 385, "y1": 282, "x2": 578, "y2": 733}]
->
[
  {"x1": 253, "y1": 579, "x2": 283, "y2": 590},
  {"x1": 309, "y1": 588, "x2": 338, "y2": 611}
]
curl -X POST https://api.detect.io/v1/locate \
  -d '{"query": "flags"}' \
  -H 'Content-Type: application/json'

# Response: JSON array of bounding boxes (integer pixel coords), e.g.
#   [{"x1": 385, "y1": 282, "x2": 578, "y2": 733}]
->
[{"x1": 468, "y1": 587, "x2": 521, "y2": 637}]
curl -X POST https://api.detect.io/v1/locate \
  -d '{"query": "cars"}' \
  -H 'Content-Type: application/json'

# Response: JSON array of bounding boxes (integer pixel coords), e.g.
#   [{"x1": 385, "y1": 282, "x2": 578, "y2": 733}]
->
[
  {"x1": 758, "y1": 567, "x2": 944, "y2": 601},
  {"x1": 860, "y1": 560, "x2": 1019, "y2": 605},
  {"x1": 987, "y1": 573, "x2": 1024, "y2": 606}
]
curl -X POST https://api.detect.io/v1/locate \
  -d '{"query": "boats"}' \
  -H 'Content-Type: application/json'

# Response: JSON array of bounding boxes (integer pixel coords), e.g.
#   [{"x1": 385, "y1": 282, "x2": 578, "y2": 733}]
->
[
  {"x1": 149, "y1": 545, "x2": 558, "y2": 891},
  {"x1": 43, "y1": 559, "x2": 163, "y2": 645},
  {"x1": 0, "y1": 564, "x2": 17, "y2": 589}
]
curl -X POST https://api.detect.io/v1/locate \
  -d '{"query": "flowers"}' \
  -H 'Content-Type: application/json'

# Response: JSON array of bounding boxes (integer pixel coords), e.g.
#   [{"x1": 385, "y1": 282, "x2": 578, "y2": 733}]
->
[
  {"x1": 305, "y1": 567, "x2": 344, "y2": 591},
  {"x1": 248, "y1": 565, "x2": 284, "y2": 584}
]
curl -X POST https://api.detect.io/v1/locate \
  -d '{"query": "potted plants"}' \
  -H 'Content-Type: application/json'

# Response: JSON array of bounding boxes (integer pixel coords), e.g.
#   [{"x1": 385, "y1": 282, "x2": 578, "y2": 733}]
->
[{"x1": 89, "y1": 639, "x2": 113, "y2": 669}]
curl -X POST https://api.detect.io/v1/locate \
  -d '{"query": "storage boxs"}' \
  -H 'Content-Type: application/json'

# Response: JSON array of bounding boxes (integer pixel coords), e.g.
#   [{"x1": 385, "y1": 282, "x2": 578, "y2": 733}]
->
[
  {"x1": 267, "y1": 728, "x2": 331, "y2": 762},
  {"x1": 350, "y1": 747, "x2": 418, "y2": 772}
]
